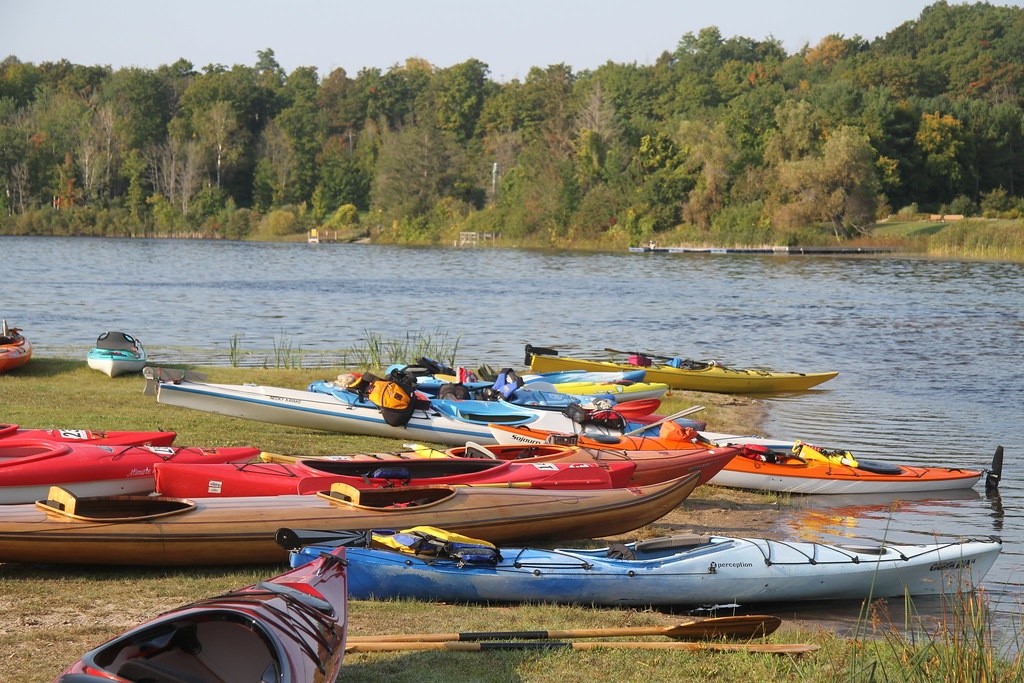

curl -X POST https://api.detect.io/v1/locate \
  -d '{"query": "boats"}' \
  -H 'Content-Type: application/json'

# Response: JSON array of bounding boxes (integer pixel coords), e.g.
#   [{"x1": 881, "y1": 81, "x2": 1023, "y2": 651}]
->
[
  {"x1": 275, "y1": 525, "x2": 1005, "y2": 612},
  {"x1": 1, "y1": 358, "x2": 1008, "y2": 560},
  {"x1": 524, "y1": 343, "x2": 840, "y2": 400},
  {"x1": 52, "y1": 545, "x2": 349, "y2": 682},
  {"x1": 0, "y1": 318, "x2": 32, "y2": 372},
  {"x1": 87, "y1": 331, "x2": 147, "y2": 379}
]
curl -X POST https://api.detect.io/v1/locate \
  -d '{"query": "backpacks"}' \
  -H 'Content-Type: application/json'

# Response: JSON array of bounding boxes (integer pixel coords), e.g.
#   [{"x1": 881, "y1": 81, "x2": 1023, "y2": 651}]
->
[{"x1": 360, "y1": 372, "x2": 417, "y2": 427}]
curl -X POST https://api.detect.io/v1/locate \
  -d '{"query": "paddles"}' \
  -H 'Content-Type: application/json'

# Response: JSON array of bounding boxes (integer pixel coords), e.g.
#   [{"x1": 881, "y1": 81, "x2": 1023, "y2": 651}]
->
[
  {"x1": 343, "y1": 615, "x2": 822, "y2": 654},
  {"x1": 604, "y1": 347, "x2": 707, "y2": 365},
  {"x1": 623, "y1": 405, "x2": 705, "y2": 435},
  {"x1": 583, "y1": 398, "x2": 661, "y2": 417}
]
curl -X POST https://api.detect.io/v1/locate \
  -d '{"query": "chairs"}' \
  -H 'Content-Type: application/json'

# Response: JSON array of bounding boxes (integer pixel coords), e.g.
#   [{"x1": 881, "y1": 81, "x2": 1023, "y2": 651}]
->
[
  {"x1": 607, "y1": 543, "x2": 636, "y2": 560},
  {"x1": 46, "y1": 486, "x2": 78, "y2": 515},
  {"x1": 441, "y1": 400, "x2": 464, "y2": 419},
  {"x1": 330, "y1": 482, "x2": 360, "y2": 504},
  {"x1": 0, "y1": 337, "x2": 12, "y2": 345},
  {"x1": 117, "y1": 658, "x2": 208, "y2": 683},
  {"x1": 660, "y1": 421, "x2": 692, "y2": 443},
  {"x1": 673, "y1": 358, "x2": 681, "y2": 368},
  {"x1": 456, "y1": 366, "x2": 471, "y2": 383},
  {"x1": 465, "y1": 441, "x2": 497, "y2": 459},
  {"x1": 97, "y1": 332, "x2": 136, "y2": 349}
]
